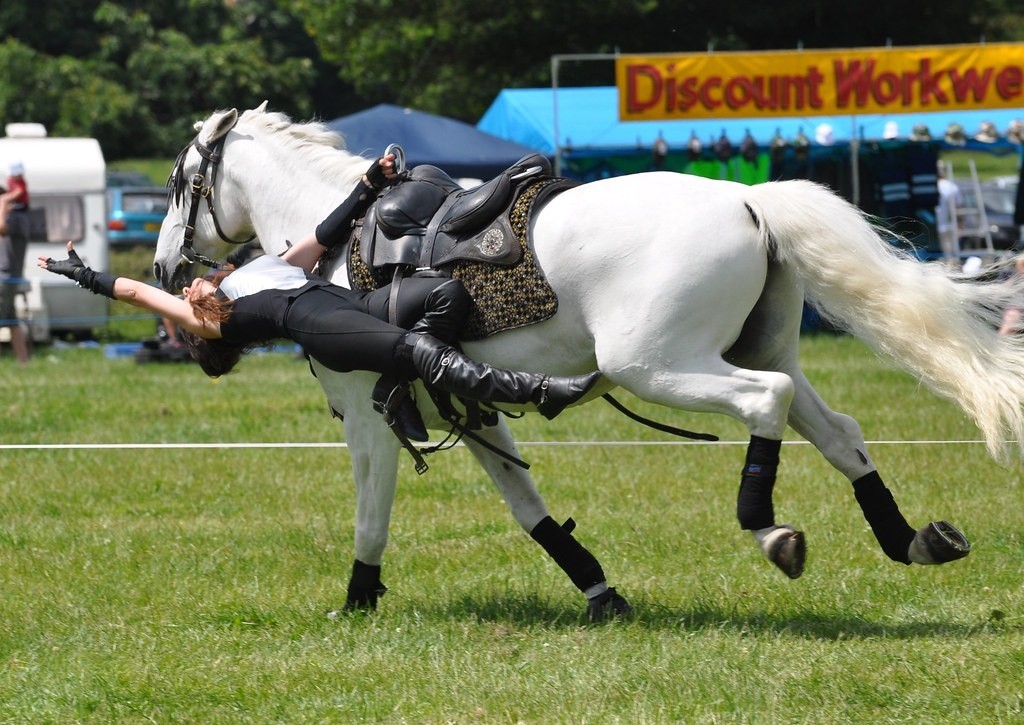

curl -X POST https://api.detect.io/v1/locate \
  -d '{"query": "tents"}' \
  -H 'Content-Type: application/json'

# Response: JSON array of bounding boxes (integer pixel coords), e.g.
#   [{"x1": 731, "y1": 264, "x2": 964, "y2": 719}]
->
[
  {"x1": 326, "y1": 103, "x2": 538, "y2": 181},
  {"x1": 474, "y1": 85, "x2": 1023, "y2": 209}
]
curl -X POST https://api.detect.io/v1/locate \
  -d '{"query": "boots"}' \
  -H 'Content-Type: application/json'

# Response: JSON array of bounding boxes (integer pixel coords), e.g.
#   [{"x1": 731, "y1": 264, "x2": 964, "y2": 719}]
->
[
  {"x1": 412, "y1": 338, "x2": 602, "y2": 420},
  {"x1": 373, "y1": 279, "x2": 476, "y2": 442}
]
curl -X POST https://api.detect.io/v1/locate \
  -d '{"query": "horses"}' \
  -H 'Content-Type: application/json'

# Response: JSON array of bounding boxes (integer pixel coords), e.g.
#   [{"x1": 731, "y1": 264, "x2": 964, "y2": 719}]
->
[{"x1": 151, "y1": 100, "x2": 1023, "y2": 628}]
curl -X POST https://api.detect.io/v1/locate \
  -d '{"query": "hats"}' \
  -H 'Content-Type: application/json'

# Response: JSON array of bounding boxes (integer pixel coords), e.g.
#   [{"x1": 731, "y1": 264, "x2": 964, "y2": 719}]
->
[{"x1": 7, "y1": 162, "x2": 24, "y2": 176}]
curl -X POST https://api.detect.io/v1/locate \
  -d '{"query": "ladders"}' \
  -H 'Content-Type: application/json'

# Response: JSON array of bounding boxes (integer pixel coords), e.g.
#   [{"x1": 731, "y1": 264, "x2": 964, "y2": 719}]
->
[{"x1": 946, "y1": 158, "x2": 994, "y2": 262}]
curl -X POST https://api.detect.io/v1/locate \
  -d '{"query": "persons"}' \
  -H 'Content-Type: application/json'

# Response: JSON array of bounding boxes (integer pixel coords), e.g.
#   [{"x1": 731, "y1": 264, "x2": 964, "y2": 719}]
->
[
  {"x1": 6, "y1": 162, "x2": 28, "y2": 211},
  {"x1": 0, "y1": 184, "x2": 31, "y2": 367},
  {"x1": 936, "y1": 159, "x2": 964, "y2": 257},
  {"x1": 36, "y1": 153, "x2": 600, "y2": 442},
  {"x1": 996, "y1": 155, "x2": 1024, "y2": 335}
]
